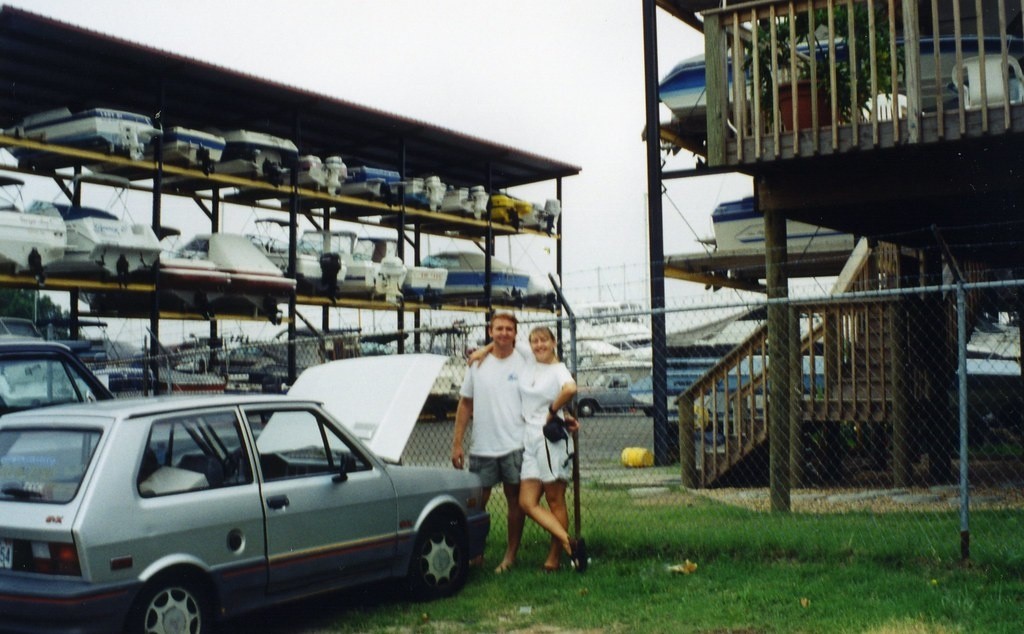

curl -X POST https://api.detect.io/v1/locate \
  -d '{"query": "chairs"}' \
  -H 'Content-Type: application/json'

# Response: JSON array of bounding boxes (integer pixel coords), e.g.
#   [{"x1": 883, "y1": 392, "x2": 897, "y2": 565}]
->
[
  {"x1": 951, "y1": 55, "x2": 1024, "y2": 111},
  {"x1": 176, "y1": 454, "x2": 224, "y2": 485}
]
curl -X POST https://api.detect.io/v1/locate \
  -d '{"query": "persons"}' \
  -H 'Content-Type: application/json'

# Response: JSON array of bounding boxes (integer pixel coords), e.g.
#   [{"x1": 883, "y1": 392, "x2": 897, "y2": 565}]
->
[
  {"x1": 468, "y1": 326, "x2": 588, "y2": 573},
  {"x1": 453, "y1": 311, "x2": 582, "y2": 573}
]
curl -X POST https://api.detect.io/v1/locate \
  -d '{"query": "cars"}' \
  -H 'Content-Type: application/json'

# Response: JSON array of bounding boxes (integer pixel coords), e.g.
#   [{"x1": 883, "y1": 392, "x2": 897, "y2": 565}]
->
[
  {"x1": 0, "y1": 352, "x2": 494, "y2": 634},
  {"x1": 1, "y1": 338, "x2": 127, "y2": 494}
]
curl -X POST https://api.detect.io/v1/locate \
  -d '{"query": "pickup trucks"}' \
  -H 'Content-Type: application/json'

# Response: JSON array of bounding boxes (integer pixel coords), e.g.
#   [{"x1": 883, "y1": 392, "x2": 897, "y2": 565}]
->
[{"x1": 575, "y1": 373, "x2": 655, "y2": 419}]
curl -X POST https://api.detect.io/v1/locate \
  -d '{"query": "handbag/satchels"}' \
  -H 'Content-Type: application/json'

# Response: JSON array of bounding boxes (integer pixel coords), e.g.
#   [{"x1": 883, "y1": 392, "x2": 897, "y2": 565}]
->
[{"x1": 543, "y1": 415, "x2": 568, "y2": 443}]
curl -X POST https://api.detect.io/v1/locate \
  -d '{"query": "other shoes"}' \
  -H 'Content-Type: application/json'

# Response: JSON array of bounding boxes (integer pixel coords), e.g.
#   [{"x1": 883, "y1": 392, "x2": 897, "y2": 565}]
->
[
  {"x1": 567, "y1": 536, "x2": 587, "y2": 572},
  {"x1": 495, "y1": 559, "x2": 514, "y2": 574},
  {"x1": 542, "y1": 561, "x2": 560, "y2": 573}
]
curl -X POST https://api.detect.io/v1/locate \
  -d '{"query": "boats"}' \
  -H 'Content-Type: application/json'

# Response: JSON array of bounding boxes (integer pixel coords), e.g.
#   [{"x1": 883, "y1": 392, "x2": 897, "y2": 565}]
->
[
  {"x1": 656, "y1": 33, "x2": 1024, "y2": 115},
  {"x1": 625, "y1": 306, "x2": 1024, "y2": 424},
  {"x1": 711, "y1": 197, "x2": 855, "y2": 256},
  {"x1": 0, "y1": 103, "x2": 560, "y2": 406}
]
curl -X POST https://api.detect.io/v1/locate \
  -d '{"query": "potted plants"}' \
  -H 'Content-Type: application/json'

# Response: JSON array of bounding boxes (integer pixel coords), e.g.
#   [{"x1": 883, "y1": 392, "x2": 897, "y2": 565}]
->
[{"x1": 741, "y1": 4, "x2": 905, "y2": 133}]
